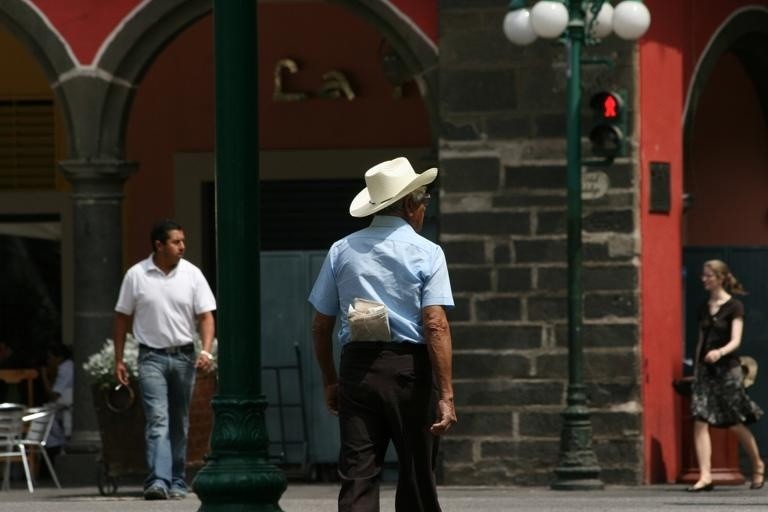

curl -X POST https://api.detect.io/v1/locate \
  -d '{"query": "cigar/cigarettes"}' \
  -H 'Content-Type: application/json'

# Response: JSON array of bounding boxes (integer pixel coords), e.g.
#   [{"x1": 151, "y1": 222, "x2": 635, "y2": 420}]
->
[{"x1": 115, "y1": 383, "x2": 123, "y2": 391}]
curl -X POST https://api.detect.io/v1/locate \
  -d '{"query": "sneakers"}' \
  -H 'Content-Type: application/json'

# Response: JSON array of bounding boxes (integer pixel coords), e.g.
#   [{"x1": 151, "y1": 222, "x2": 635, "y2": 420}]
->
[
  {"x1": 169, "y1": 479, "x2": 186, "y2": 500},
  {"x1": 144, "y1": 480, "x2": 169, "y2": 499}
]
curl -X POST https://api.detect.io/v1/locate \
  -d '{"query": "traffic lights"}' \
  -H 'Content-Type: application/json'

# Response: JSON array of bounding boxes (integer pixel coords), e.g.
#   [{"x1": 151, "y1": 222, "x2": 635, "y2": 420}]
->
[{"x1": 590, "y1": 91, "x2": 620, "y2": 156}]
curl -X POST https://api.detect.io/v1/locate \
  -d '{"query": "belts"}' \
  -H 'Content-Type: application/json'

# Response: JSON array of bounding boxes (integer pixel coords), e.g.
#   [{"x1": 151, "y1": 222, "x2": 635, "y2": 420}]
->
[
  {"x1": 346, "y1": 341, "x2": 418, "y2": 354},
  {"x1": 138, "y1": 341, "x2": 194, "y2": 355}
]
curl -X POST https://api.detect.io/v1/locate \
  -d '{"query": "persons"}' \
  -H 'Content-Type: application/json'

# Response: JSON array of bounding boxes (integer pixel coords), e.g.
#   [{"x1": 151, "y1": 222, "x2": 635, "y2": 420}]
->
[
  {"x1": 41, "y1": 345, "x2": 74, "y2": 437},
  {"x1": 308, "y1": 156, "x2": 457, "y2": 512},
  {"x1": 684, "y1": 260, "x2": 764, "y2": 492},
  {"x1": 114, "y1": 219, "x2": 216, "y2": 500}
]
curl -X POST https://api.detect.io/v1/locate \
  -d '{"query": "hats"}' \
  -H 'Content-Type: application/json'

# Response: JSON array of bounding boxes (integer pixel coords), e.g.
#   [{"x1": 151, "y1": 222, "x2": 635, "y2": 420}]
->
[{"x1": 348, "y1": 155, "x2": 440, "y2": 219}]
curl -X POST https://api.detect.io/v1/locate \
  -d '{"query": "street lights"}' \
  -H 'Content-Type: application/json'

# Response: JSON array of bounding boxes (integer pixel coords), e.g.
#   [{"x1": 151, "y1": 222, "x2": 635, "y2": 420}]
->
[{"x1": 504, "y1": 1, "x2": 652, "y2": 493}]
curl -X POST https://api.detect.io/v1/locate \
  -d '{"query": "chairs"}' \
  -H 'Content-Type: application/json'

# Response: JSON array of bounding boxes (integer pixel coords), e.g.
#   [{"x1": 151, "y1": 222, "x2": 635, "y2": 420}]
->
[{"x1": 0, "y1": 402, "x2": 67, "y2": 495}]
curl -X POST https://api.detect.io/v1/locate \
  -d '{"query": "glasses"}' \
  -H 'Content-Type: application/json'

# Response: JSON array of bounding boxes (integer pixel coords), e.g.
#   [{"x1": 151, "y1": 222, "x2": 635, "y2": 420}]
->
[{"x1": 423, "y1": 192, "x2": 431, "y2": 206}]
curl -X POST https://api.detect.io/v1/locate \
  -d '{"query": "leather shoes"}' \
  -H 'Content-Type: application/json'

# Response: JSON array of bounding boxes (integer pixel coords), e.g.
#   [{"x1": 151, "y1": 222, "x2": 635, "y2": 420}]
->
[
  {"x1": 750, "y1": 471, "x2": 765, "y2": 490},
  {"x1": 686, "y1": 482, "x2": 715, "y2": 492}
]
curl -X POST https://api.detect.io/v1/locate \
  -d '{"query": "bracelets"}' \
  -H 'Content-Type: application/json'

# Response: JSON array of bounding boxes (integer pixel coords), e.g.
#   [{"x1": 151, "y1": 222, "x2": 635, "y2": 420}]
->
[
  {"x1": 199, "y1": 350, "x2": 214, "y2": 360},
  {"x1": 717, "y1": 348, "x2": 724, "y2": 357}
]
